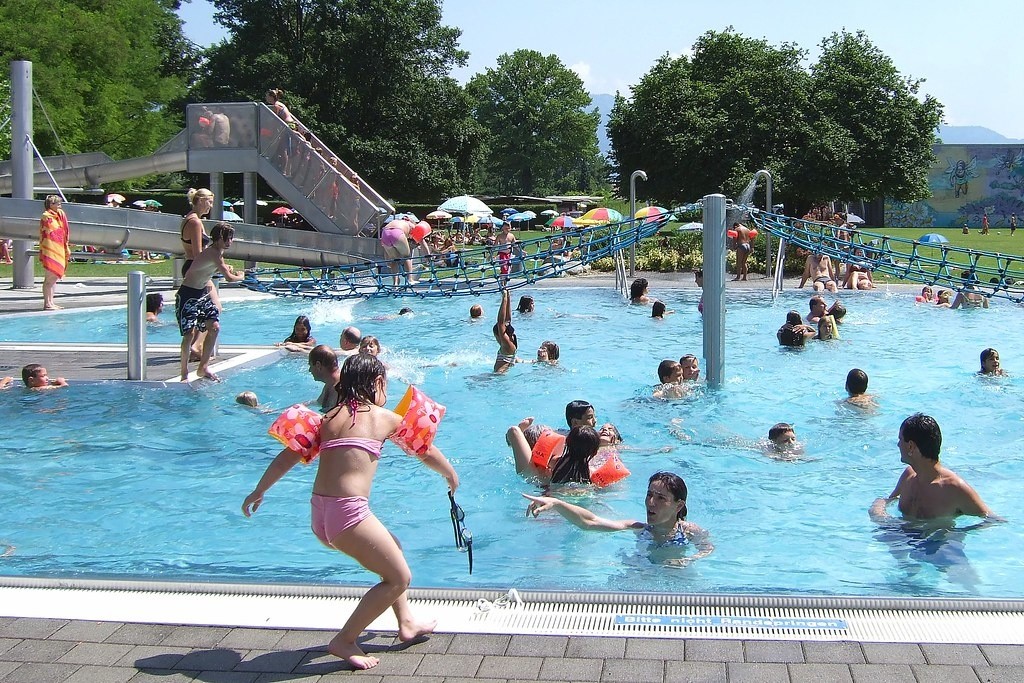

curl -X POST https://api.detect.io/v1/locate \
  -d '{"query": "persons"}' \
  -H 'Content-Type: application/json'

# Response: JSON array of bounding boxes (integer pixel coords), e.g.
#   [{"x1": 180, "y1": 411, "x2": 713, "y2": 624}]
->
[
  {"x1": 382, "y1": 216, "x2": 431, "y2": 289},
  {"x1": 776, "y1": 212, "x2": 876, "y2": 293},
  {"x1": 0, "y1": 364, "x2": 68, "y2": 391},
  {"x1": 266, "y1": 89, "x2": 325, "y2": 197},
  {"x1": 962, "y1": 214, "x2": 989, "y2": 236},
  {"x1": 1010, "y1": 213, "x2": 1017, "y2": 237},
  {"x1": 147, "y1": 188, "x2": 244, "y2": 381},
  {"x1": 493, "y1": 222, "x2": 527, "y2": 286},
  {"x1": 39, "y1": 196, "x2": 71, "y2": 309},
  {"x1": 241, "y1": 354, "x2": 460, "y2": 671},
  {"x1": 325, "y1": 157, "x2": 360, "y2": 236},
  {"x1": 237, "y1": 271, "x2": 1009, "y2": 534},
  {"x1": 0, "y1": 239, "x2": 14, "y2": 265},
  {"x1": 191, "y1": 105, "x2": 231, "y2": 147},
  {"x1": 91, "y1": 198, "x2": 161, "y2": 260},
  {"x1": 731, "y1": 222, "x2": 754, "y2": 281},
  {"x1": 426, "y1": 229, "x2": 496, "y2": 267}
]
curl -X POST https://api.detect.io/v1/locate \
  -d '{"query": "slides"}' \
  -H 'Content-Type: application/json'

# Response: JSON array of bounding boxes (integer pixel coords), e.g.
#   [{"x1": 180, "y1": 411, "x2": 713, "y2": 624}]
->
[{"x1": 0, "y1": 128, "x2": 386, "y2": 272}]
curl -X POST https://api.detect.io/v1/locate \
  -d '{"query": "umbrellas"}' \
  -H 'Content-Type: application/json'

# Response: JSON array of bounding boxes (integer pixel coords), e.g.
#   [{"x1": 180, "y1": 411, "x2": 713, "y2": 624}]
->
[
  {"x1": 426, "y1": 193, "x2": 537, "y2": 231},
  {"x1": 107, "y1": 194, "x2": 163, "y2": 207},
  {"x1": 222, "y1": 199, "x2": 300, "y2": 224},
  {"x1": 847, "y1": 214, "x2": 865, "y2": 225},
  {"x1": 918, "y1": 232, "x2": 950, "y2": 256},
  {"x1": 540, "y1": 206, "x2": 704, "y2": 241}
]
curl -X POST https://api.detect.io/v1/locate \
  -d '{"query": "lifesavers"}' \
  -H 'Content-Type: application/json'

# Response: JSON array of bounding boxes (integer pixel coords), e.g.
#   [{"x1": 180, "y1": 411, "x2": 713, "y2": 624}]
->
[
  {"x1": 728, "y1": 229, "x2": 758, "y2": 239},
  {"x1": 268, "y1": 385, "x2": 447, "y2": 465},
  {"x1": 532, "y1": 428, "x2": 632, "y2": 486},
  {"x1": 408, "y1": 221, "x2": 432, "y2": 244},
  {"x1": 916, "y1": 293, "x2": 939, "y2": 302},
  {"x1": 828, "y1": 315, "x2": 839, "y2": 336}
]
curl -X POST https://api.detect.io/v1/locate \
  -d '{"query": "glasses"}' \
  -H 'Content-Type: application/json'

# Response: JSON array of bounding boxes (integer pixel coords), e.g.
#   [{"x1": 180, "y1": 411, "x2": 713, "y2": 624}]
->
[{"x1": 452, "y1": 503, "x2": 472, "y2": 546}]
